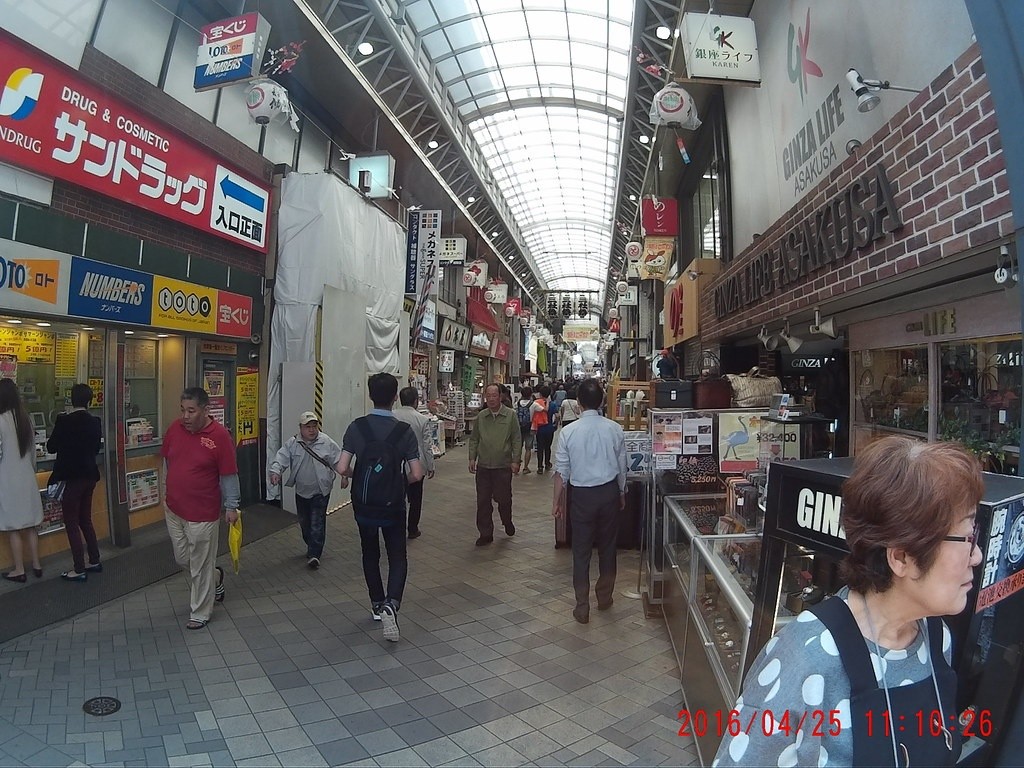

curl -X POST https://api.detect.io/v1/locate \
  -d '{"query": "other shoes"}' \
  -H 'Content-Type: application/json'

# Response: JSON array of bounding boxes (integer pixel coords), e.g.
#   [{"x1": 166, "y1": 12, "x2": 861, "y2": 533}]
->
[
  {"x1": 408, "y1": 531, "x2": 421, "y2": 539},
  {"x1": 505, "y1": 522, "x2": 515, "y2": 536},
  {"x1": 573, "y1": 610, "x2": 589, "y2": 623},
  {"x1": 517, "y1": 464, "x2": 552, "y2": 474},
  {"x1": 476, "y1": 535, "x2": 493, "y2": 546},
  {"x1": 597, "y1": 597, "x2": 613, "y2": 610},
  {"x1": 308, "y1": 555, "x2": 321, "y2": 567}
]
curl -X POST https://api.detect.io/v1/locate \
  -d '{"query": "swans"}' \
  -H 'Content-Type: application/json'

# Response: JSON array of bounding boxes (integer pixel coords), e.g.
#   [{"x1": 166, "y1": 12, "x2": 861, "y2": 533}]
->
[{"x1": 721, "y1": 416, "x2": 749, "y2": 461}]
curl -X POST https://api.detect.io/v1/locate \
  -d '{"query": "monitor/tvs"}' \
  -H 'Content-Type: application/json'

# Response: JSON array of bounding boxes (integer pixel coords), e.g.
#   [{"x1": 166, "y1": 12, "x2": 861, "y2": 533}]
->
[{"x1": 30, "y1": 413, "x2": 46, "y2": 428}]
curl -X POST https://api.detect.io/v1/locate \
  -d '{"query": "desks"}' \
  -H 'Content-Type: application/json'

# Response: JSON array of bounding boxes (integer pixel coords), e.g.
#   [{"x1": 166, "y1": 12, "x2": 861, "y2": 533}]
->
[{"x1": 444, "y1": 421, "x2": 456, "y2": 449}]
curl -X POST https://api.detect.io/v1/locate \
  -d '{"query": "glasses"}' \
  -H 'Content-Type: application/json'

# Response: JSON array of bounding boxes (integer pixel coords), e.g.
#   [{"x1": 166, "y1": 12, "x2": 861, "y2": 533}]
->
[{"x1": 939, "y1": 524, "x2": 980, "y2": 557}]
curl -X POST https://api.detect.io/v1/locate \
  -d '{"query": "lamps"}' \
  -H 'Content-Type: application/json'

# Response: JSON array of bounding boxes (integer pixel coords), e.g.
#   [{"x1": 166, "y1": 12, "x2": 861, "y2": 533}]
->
[
  {"x1": 756, "y1": 324, "x2": 779, "y2": 351},
  {"x1": 687, "y1": 270, "x2": 718, "y2": 281},
  {"x1": 845, "y1": 68, "x2": 923, "y2": 113},
  {"x1": 810, "y1": 306, "x2": 839, "y2": 339},
  {"x1": 993, "y1": 244, "x2": 1019, "y2": 289},
  {"x1": 779, "y1": 318, "x2": 805, "y2": 354}
]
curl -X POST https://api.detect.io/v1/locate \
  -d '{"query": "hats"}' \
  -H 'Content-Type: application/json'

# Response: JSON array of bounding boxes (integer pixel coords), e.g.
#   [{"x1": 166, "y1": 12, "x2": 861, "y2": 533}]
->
[{"x1": 298, "y1": 412, "x2": 318, "y2": 426}]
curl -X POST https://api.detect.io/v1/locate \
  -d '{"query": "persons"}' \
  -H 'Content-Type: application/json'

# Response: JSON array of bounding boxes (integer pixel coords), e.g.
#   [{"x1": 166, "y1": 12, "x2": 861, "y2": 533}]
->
[
  {"x1": 393, "y1": 387, "x2": 436, "y2": 540},
  {"x1": 269, "y1": 411, "x2": 349, "y2": 569},
  {"x1": 337, "y1": 372, "x2": 423, "y2": 642},
  {"x1": 46, "y1": 384, "x2": 103, "y2": 582},
  {"x1": 552, "y1": 378, "x2": 629, "y2": 624},
  {"x1": 478, "y1": 377, "x2": 644, "y2": 474},
  {"x1": 468, "y1": 383, "x2": 522, "y2": 546},
  {"x1": 160, "y1": 387, "x2": 242, "y2": 630},
  {"x1": 709, "y1": 435, "x2": 982, "y2": 768},
  {"x1": 0, "y1": 378, "x2": 44, "y2": 582}
]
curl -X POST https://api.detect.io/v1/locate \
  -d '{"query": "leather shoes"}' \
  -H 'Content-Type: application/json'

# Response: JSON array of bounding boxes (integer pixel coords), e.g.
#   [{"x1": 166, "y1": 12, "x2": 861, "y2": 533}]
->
[
  {"x1": 84, "y1": 562, "x2": 103, "y2": 572},
  {"x1": 61, "y1": 570, "x2": 88, "y2": 582}
]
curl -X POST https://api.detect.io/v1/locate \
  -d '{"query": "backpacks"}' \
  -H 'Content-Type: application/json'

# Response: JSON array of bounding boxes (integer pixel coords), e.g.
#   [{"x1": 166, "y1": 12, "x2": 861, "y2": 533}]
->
[
  {"x1": 517, "y1": 399, "x2": 534, "y2": 433},
  {"x1": 352, "y1": 416, "x2": 411, "y2": 526}
]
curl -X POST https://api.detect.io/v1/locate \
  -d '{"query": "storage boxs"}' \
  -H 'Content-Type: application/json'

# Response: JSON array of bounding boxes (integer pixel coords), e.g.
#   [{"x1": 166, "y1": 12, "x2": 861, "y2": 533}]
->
[{"x1": 755, "y1": 412, "x2": 837, "y2": 541}]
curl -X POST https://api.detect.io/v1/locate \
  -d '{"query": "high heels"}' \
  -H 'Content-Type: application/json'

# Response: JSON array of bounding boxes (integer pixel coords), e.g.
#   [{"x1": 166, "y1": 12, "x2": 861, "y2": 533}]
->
[
  {"x1": 1, "y1": 573, "x2": 26, "y2": 583},
  {"x1": 33, "y1": 568, "x2": 42, "y2": 578}
]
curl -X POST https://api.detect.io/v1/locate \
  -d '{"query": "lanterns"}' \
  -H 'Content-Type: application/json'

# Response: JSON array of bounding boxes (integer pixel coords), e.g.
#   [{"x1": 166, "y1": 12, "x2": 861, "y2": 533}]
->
[
  {"x1": 600, "y1": 279, "x2": 629, "y2": 352},
  {"x1": 484, "y1": 288, "x2": 496, "y2": 304},
  {"x1": 653, "y1": 81, "x2": 693, "y2": 129},
  {"x1": 247, "y1": 82, "x2": 283, "y2": 124},
  {"x1": 505, "y1": 306, "x2": 515, "y2": 318},
  {"x1": 463, "y1": 269, "x2": 477, "y2": 285},
  {"x1": 520, "y1": 316, "x2": 558, "y2": 350},
  {"x1": 625, "y1": 240, "x2": 643, "y2": 263}
]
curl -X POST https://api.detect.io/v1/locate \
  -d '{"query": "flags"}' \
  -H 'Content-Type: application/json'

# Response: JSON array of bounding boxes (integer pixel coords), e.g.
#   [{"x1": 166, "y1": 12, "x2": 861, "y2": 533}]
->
[{"x1": 411, "y1": 264, "x2": 433, "y2": 350}]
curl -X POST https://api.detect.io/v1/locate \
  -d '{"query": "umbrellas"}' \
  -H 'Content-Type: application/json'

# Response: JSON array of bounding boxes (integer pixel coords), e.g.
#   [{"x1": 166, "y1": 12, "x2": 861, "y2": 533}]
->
[{"x1": 522, "y1": 372, "x2": 539, "y2": 380}]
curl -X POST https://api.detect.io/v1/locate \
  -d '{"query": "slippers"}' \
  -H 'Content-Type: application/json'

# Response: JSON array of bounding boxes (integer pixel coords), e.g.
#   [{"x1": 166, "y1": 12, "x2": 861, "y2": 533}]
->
[
  {"x1": 215, "y1": 567, "x2": 225, "y2": 602},
  {"x1": 186, "y1": 619, "x2": 207, "y2": 629}
]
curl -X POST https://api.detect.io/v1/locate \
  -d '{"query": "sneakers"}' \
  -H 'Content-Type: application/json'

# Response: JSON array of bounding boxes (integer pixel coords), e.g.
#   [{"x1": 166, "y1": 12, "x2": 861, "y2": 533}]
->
[
  {"x1": 371, "y1": 605, "x2": 381, "y2": 621},
  {"x1": 380, "y1": 602, "x2": 400, "y2": 641}
]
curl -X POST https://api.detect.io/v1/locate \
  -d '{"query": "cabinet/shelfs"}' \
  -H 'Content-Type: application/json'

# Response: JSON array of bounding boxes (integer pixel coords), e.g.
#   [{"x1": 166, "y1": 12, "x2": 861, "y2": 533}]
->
[{"x1": 429, "y1": 420, "x2": 446, "y2": 459}]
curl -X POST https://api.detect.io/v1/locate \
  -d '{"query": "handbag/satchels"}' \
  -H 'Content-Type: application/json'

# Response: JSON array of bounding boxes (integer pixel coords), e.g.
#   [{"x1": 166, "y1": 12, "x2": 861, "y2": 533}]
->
[
  {"x1": 801, "y1": 393, "x2": 816, "y2": 413},
  {"x1": 652, "y1": 348, "x2": 782, "y2": 408},
  {"x1": 47, "y1": 480, "x2": 67, "y2": 502},
  {"x1": 228, "y1": 509, "x2": 242, "y2": 575}
]
